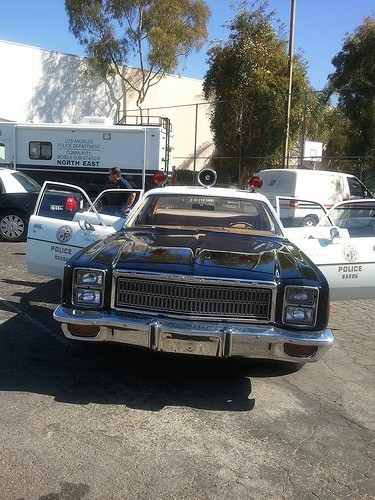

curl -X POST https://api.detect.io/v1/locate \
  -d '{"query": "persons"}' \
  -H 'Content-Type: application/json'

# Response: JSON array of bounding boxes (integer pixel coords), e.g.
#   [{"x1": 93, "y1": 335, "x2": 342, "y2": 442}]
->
[{"x1": 108, "y1": 166, "x2": 137, "y2": 218}]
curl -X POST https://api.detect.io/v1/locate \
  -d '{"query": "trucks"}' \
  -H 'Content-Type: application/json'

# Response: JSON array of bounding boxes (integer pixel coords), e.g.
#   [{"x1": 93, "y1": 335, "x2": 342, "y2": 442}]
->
[{"x1": 0, "y1": 122, "x2": 165, "y2": 212}]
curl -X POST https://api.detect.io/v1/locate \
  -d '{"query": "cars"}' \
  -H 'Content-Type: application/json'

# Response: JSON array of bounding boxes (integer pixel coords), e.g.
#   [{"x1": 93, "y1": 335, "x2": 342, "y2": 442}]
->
[
  {"x1": 26, "y1": 179, "x2": 375, "y2": 373},
  {"x1": 0, "y1": 167, "x2": 84, "y2": 242}
]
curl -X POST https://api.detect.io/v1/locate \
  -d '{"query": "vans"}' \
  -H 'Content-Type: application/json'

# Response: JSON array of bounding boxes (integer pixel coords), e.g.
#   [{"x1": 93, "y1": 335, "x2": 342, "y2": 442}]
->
[{"x1": 254, "y1": 169, "x2": 375, "y2": 231}]
